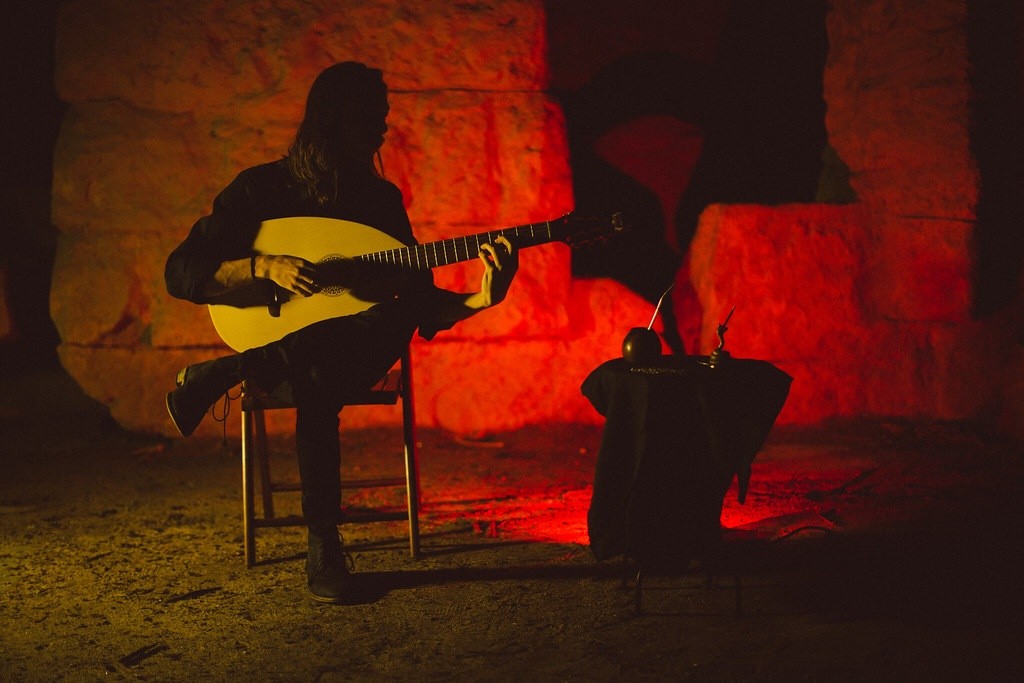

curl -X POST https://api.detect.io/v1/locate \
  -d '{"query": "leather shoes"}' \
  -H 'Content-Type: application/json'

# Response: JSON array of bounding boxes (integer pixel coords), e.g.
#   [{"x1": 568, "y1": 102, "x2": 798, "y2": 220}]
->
[
  {"x1": 166, "y1": 353, "x2": 245, "y2": 439},
  {"x1": 305, "y1": 528, "x2": 353, "y2": 602}
]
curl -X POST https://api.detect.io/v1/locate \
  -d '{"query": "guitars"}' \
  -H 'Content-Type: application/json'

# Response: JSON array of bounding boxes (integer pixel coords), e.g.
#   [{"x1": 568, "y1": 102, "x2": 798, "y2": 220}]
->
[{"x1": 206, "y1": 208, "x2": 633, "y2": 354}]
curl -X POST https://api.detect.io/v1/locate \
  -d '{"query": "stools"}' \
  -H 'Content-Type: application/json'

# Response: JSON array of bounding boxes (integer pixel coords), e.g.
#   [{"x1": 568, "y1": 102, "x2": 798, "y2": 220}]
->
[{"x1": 580, "y1": 355, "x2": 792, "y2": 611}]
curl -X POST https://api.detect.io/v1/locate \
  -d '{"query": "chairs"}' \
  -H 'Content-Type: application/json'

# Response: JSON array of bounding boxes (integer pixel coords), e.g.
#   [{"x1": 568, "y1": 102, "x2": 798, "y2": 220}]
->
[{"x1": 238, "y1": 347, "x2": 422, "y2": 568}]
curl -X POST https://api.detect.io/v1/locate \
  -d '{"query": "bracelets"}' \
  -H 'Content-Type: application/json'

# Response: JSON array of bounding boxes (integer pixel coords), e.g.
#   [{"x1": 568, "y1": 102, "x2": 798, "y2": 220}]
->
[{"x1": 251, "y1": 255, "x2": 255, "y2": 280}]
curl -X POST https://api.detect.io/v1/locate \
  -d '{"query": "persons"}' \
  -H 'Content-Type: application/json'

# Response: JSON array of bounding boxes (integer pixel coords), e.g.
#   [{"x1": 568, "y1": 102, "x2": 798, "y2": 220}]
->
[{"x1": 164, "y1": 62, "x2": 518, "y2": 603}]
